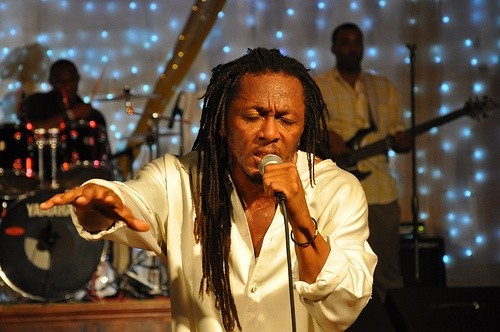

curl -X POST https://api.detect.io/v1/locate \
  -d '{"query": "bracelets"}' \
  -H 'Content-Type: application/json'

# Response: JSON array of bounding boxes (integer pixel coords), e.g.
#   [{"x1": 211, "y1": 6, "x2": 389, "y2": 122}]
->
[
  {"x1": 290, "y1": 217, "x2": 318, "y2": 245},
  {"x1": 67, "y1": 109, "x2": 74, "y2": 121}
]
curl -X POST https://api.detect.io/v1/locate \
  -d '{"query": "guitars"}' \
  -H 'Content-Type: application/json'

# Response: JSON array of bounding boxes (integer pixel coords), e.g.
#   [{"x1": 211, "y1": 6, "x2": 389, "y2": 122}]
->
[{"x1": 324, "y1": 94, "x2": 499, "y2": 181}]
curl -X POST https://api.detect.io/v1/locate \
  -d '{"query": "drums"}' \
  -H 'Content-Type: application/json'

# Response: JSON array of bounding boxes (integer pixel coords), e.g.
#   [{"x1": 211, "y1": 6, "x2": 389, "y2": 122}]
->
[
  {"x1": 0, "y1": 189, "x2": 108, "y2": 302},
  {"x1": 43, "y1": 131, "x2": 91, "y2": 179},
  {"x1": 0, "y1": 123, "x2": 40, "y2": 195},
  {"x1": 399, "y1": 222, "x2": 425, "y2": 241},
  {"x1": 399, "y1": 236, "x2": 447, "y2": 304}
]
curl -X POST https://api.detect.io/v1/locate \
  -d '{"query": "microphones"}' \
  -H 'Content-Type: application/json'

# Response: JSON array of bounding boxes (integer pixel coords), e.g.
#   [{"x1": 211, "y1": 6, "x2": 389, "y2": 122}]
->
[
  {"x1": 169, "y1": 92, "x2": 181, "y2": 128},
  {"x1": 258, "y1": 154, "x2": 286, "y2": 203}
]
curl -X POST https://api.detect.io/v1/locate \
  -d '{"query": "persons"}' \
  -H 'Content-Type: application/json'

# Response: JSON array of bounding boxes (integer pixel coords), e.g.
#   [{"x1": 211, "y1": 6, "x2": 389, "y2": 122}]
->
[
  {"x1": 311, "y1": 22, "x2": 413, "y2": 303},
  {"x1": 39, "y1": 47, "x2": 379, "y2": 332},
  {"x1": 18, "y1": 59, "x2": 112, "y2": 179}
]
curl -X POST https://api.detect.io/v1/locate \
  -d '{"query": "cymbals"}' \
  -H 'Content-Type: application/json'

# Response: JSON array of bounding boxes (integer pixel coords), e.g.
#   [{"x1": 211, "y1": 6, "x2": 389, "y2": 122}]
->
[
  {"x1": 122, "y1": 133, "x2": 177, "y2": 140},
  {"x1": 96, "y1": 93, "x2": 162, "y2": 101}
]
